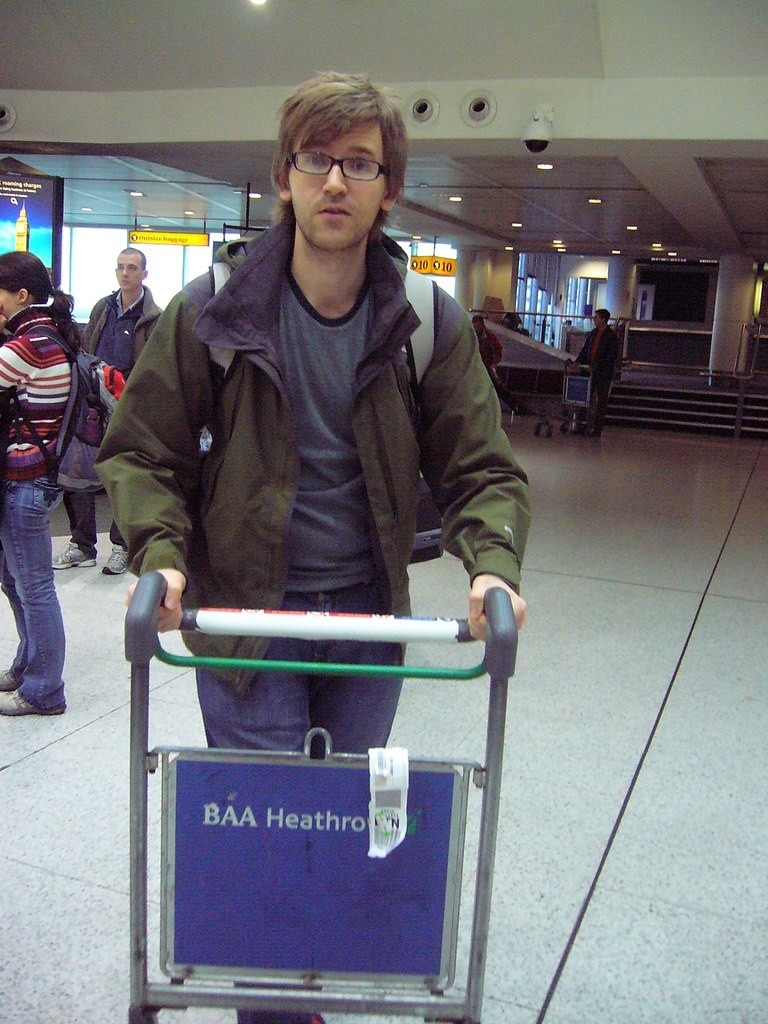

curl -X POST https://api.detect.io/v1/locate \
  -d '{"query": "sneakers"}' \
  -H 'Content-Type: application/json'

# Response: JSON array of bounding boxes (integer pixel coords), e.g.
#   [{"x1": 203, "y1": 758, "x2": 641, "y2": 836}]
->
[
  {"x1": 52, "y1": 542, "x2": 97, "y2": 569},
  {"x1": 0, "y1": 669, "x2": 20, "y2": 691},
  {"x1": 102, "y1": 544, "x2": 129, "y2": 574},
  {"x1": 0, "y1": 688, "x2": 65, "y2": 716}
]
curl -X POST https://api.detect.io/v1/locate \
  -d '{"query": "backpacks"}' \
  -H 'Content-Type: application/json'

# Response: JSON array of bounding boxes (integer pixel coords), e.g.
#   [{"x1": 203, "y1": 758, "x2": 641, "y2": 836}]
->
[{"x1": 16, "y1": 326, "x2": 125, "y2": 492}]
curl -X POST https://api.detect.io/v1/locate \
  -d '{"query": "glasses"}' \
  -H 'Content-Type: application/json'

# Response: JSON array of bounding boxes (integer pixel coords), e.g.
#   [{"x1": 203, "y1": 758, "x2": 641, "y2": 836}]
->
[{"x1": 287, "y1": 152, "x2": 389, "y2": 181}]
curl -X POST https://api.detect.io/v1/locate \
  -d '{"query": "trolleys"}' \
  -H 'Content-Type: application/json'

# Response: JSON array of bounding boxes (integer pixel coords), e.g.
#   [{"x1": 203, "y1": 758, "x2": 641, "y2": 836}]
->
[
  {"x1": 533, "y1": 364, "x2": 599, "y2": 440},
  {"x1": 123, "y1": 570, "x2": 518, "y2": 1023}
]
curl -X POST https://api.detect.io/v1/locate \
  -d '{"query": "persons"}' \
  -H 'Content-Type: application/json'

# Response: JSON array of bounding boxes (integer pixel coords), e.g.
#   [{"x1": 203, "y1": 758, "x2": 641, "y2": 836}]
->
[
  {"x1": 92, "y1": 72, "x2": 532, "y2": 1024},
  {"x1": 570, "y1": 309, "x2": 620, "y2": 437},
  {"x1": 0, "y1": 251, "x2": 81, "y2": 716},
  {"x1": 472, "y1": 315, "x2": 502, "y2": 375},
  {"x1": 52, "y1": 248, "x2": 165, "y2": 575}
]
